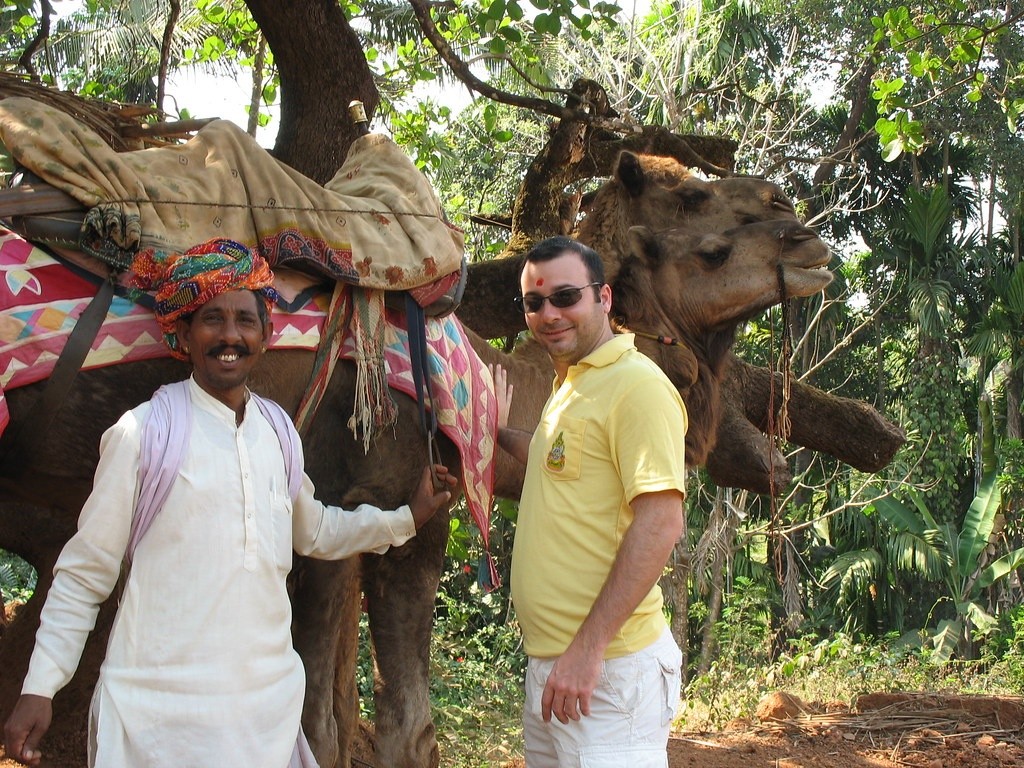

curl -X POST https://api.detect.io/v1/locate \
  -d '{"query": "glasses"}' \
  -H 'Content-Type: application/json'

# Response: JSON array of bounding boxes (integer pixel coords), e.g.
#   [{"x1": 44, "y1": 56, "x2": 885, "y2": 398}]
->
[{"x1": 514, "y1": 281, "x2": 604, "y2": 312}]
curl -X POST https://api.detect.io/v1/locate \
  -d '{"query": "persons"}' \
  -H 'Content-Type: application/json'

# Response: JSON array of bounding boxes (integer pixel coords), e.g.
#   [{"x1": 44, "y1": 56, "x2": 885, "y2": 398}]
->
[
  {"x1": 488, "y1": 236, "x2": 688, "y2": 768},
  {"x1": 4, "y1": 239, "x2": 457, "y2": 768}
]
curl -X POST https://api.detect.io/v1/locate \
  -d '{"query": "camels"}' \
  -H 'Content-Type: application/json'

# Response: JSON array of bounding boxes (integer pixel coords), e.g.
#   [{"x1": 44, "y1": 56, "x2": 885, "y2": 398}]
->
[{"x1": 0, "y1": 147, "x2": 912, "y2": 768}]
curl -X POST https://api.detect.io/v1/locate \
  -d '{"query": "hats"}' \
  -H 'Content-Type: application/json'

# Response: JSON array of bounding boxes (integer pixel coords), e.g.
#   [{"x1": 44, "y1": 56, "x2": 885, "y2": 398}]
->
[{"x1": 131, "y1": 238, "x2": 280, "y2": 360}]
